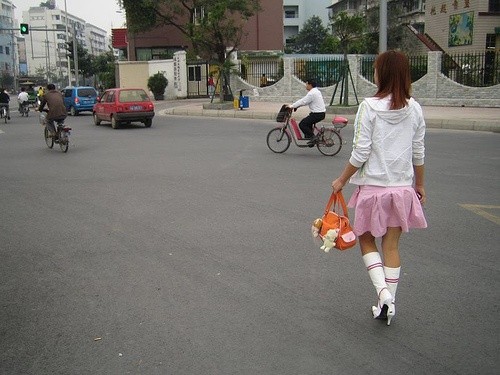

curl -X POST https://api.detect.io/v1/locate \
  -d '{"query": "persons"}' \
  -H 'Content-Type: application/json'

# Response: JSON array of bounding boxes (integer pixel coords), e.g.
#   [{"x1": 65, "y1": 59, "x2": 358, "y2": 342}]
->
[
  {"x1": 284, "y1": 80, "x2": 326, "y2": 148},
  {"x1": 260, "y1": 74, "x2": 267, "y2": 88},
  {"x1": 38, "y1": 87, "x2": 43, "y2": 97},
  {"x1": 0, "y1": 88, "x2": 11, "y2": 120},
  {"x1": 208, "y1": 75, "x2": 215, "y2": 98},
  {"x1": 17, "y1": 88, "x2": 29, "y2": 113},
  {"x1": 332, "y1": 51, "x2": 427, "y2": 325},
  {"x1": 36, "y1": 84, "x2": 67, "y2": 143}
]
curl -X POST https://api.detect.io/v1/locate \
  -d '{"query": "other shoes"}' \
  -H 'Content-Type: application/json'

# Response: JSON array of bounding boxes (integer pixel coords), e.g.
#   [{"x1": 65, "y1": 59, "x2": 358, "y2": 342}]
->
[
  {"x1": 8, "y1": 117, "x2": 11, "y2": 120},
  {"x1": 307, "y1": 137, "x2": 317, "y2": 144},
  {"x1": 50, "y1": 133, "x2": 55, "y2": 137}
]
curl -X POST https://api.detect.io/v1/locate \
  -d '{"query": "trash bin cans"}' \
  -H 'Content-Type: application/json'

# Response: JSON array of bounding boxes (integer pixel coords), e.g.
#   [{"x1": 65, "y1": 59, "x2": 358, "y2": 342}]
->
[{"x1": 233, "y1": 89, "x2": 250, "y2": 110}]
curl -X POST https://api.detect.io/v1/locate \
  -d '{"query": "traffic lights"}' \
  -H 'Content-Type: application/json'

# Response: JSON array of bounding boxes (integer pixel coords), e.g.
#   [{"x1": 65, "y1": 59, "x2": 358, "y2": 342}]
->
[
  {"x1": 20, "y1": 23, "x2": 29, "y2": 34},
  {"x1": 66, "y1": 40, "x2": 75, "y2": 60}
]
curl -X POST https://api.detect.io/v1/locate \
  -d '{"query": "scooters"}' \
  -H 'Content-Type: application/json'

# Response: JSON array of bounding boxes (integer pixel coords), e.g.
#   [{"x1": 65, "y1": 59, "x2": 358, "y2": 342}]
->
[{"x1": 266, "y1": 104, "x2": 349, "y2": 157}]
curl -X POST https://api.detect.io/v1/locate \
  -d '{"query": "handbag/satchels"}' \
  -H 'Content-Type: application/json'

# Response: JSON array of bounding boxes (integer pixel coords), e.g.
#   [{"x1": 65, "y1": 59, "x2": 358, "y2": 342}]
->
[
  {"x1": 275, "y1": 104, "x2": 288, "y2": 121},
  {"x1": 311, "y1": 191, "x2": 356, "y2": 253}
]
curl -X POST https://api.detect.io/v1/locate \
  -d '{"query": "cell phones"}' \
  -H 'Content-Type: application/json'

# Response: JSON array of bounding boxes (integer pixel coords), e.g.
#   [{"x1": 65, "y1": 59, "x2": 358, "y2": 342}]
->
[{"x1": 416, "y1": 192, "x2": 421, "y2": 200}]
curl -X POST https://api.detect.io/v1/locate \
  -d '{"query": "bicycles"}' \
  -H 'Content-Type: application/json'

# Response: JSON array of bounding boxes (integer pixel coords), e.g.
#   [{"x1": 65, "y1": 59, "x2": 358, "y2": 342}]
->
[
  {"x1": 41, "y1": 108, "x2": 72, "y2": 153},
  {"x1": 1, "y1": 105, "x2": 9, "y2": 123},
  {"x1": 18, "y1": 98, "x2": 30, "y2": 117}
]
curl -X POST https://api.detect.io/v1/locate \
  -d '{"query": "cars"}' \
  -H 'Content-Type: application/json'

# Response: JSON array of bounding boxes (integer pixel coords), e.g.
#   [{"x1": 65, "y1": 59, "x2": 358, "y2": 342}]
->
[
  {"x1": 92, "y1": 87, "x2": 156, "y2": 129},
  {"x1": 27, "y1": 91, "x2": 37, "y2": 103},
  {"x1": 60, "y1": 86, "x2": 98, "y2": 116}
]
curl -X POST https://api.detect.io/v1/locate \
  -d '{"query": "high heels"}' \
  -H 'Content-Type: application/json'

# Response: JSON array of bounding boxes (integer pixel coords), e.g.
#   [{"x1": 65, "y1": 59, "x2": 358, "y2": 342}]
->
[
  {"x1": 386, "y1": 304, "x2": 395, "y2": 325},
  {"x1": 371, "y1": 290, "x2": 394, "y2": 323}
]
